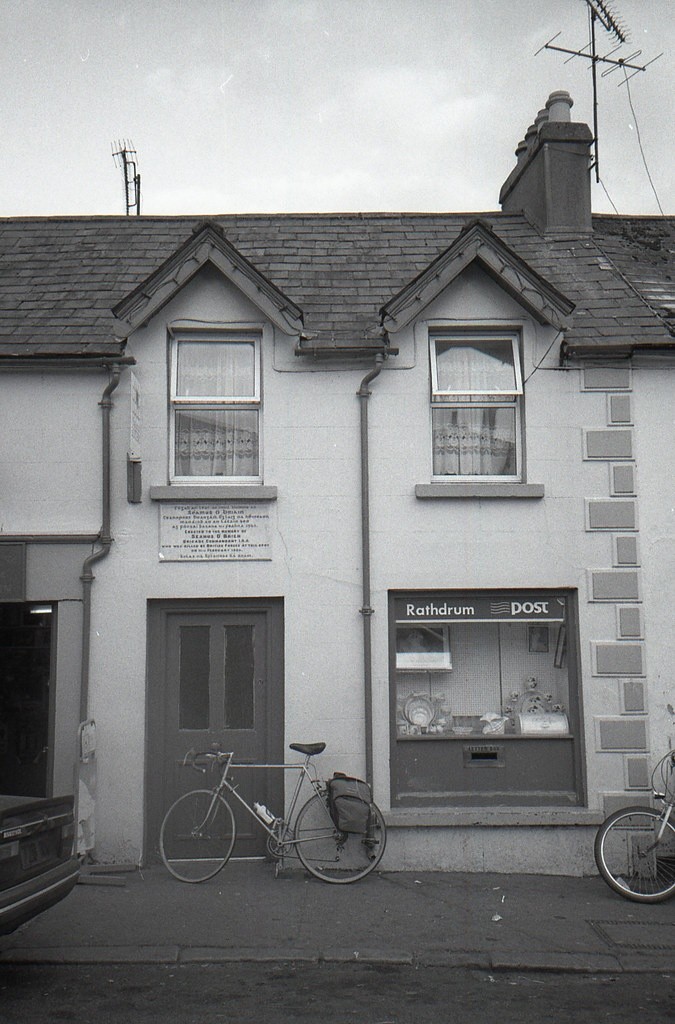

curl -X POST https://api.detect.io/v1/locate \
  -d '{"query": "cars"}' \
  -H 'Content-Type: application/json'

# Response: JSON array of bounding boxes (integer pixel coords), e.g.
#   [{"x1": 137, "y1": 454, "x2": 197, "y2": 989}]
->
[{"x1": 0, "y1": 793, "x2": 82, "y2": 937}]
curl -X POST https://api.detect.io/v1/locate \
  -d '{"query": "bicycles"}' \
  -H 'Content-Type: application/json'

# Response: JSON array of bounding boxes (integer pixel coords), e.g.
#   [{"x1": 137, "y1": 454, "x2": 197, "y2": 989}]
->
[
  {"x1": 594, "y1": 703, "x2": 675, "y2": 904},
  {"x1": 159, "y1": 742, "x2": 387, "y2": 884}
]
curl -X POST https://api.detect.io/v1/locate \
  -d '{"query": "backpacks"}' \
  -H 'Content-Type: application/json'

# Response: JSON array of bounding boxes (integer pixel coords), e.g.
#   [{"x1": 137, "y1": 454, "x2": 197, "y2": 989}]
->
[{"x1": 329, "y1": 776, "x2": 372, "y2": 835}]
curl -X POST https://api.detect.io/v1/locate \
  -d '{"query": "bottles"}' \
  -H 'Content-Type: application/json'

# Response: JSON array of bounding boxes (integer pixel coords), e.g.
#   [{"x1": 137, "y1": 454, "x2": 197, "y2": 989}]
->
[{"x1": 253, "y1": 802, "x2": 275, "y2": 825}]
